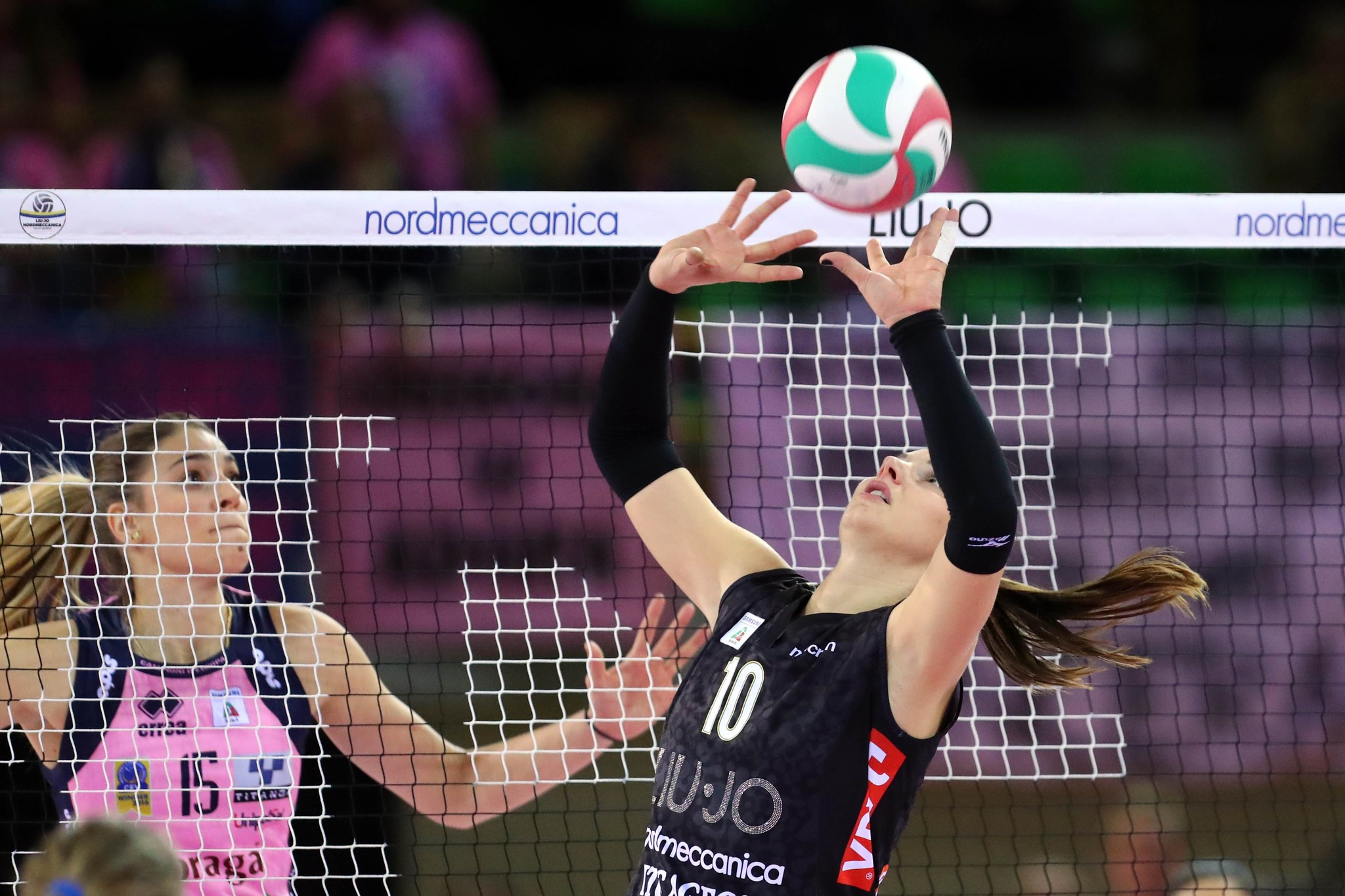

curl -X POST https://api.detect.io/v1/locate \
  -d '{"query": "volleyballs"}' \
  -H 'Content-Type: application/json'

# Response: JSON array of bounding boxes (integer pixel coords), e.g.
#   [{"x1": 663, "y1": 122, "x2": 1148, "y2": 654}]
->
[{"x1": 782, "y1": 45, "x2": 952, "y2": 212}]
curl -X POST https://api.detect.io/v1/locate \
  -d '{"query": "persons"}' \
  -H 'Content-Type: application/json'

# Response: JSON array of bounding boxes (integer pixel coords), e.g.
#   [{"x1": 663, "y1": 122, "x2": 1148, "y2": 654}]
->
[
  {"x1": 0, "y1": 410, "x2": 709, "y2": 896},
  {"x1": 586, "y1": 174, "x2": 1211, "y2": 894}
]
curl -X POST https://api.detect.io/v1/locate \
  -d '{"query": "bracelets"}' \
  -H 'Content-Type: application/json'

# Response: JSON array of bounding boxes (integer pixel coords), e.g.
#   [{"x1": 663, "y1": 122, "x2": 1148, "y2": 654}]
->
[{"x1": 583, "y1": 703, "x2": 625, "y2": 748}]
